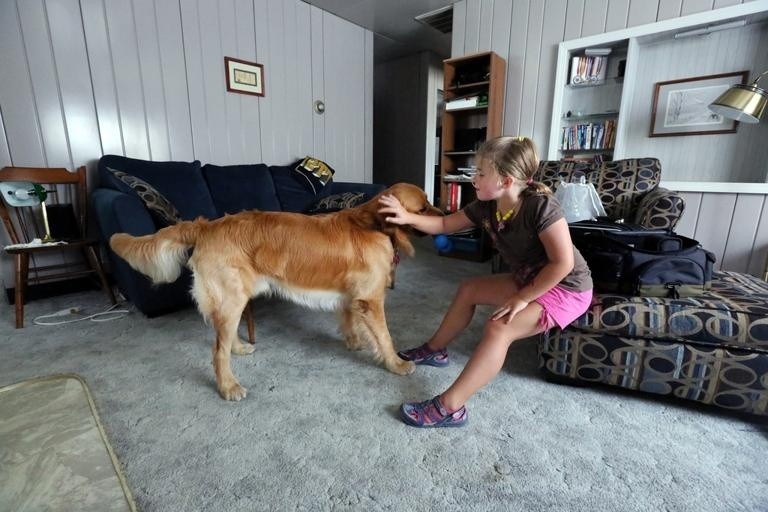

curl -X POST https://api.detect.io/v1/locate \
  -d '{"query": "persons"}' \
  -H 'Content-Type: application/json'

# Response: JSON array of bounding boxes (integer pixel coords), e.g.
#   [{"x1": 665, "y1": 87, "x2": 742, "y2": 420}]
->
[{"x1": 378, "y1": 135, "x2": 594, "y2": 428}]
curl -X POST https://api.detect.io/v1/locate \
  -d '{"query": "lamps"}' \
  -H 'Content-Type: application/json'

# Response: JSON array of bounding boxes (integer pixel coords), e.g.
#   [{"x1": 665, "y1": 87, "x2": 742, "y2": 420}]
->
[
  {"x1": 0, "y1": 180, "x2": 57, "y2": 244},
  {"x1": 708, "y1": 71, "x2": 768, "y2": 124}
]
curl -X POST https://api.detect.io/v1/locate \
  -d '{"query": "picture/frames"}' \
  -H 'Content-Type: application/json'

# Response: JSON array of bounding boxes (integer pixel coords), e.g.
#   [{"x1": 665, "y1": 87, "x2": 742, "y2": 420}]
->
[
  {"x1": 224, "y1": 56, "x2": 265, "y2": 97},
  {"x1": 649, "y1": 70, "x2": 750, "y2": 137}
]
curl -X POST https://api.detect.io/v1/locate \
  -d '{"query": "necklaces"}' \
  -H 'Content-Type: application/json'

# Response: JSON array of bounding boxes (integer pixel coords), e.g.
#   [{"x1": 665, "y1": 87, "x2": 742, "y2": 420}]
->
[{"x1": 496, "y1": 198, "x2": 521, "y2": 232}]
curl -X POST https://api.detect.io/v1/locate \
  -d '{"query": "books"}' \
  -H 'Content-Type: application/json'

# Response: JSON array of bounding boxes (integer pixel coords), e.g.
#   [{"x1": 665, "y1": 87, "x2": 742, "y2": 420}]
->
[
  {"x1": 443, "y1": 167, "x2": 478, "y2": 213},
  {"x1": 562, "y1": 120, "x2": 617, "y2": 151},
  {"x1": 570, "y1": 56, "x2": 607, "y2": 81}
]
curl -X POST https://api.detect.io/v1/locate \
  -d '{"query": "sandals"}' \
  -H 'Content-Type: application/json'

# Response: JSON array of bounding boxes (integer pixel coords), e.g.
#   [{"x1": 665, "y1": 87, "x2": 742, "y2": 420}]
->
[
  {"x1": 399, "y1": 343, "x2": 449, "y2": 367},
  {"x1": 400, "y1": 395, "x2": 468, "y2": 427}
]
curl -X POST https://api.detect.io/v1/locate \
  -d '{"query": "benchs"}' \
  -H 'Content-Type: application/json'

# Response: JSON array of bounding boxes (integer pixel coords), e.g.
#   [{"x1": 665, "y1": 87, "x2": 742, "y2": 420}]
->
[{"x1": 536, "y1": 270, "x2": 768, "y2": 415}]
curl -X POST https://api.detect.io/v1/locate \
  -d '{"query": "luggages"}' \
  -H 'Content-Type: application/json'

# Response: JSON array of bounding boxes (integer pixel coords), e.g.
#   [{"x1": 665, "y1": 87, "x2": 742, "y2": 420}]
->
[
  {"x1": 568, "y1": 216, "x2": 716, "y2": 299},
  {"x1": 433, "y1": 227, "x2": 493, "y2": 262}
]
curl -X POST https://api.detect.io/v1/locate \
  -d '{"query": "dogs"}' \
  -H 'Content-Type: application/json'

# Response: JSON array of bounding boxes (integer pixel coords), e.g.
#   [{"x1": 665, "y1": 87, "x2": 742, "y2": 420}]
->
[{"x1": 108, "y1": 182, "x2": 446, "y2": 403}]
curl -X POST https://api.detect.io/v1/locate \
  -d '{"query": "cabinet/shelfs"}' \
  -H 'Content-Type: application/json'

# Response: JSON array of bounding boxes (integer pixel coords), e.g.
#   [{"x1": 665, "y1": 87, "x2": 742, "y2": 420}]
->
[
  {"x1": 437, "y1": 51, "x2": 506, "y2": 263},
  {"x1": 548, "y1": 38, "x2": 641, "y2": 161}
]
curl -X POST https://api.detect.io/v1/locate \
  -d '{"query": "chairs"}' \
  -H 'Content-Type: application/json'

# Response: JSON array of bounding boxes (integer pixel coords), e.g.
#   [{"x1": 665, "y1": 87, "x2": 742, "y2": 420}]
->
[
  {"x1": 533, "y1": 158, "x2": 686, "y2": 232},
  {"x1": 0, "y1": 166, "x2": 119, "y2": 329}
]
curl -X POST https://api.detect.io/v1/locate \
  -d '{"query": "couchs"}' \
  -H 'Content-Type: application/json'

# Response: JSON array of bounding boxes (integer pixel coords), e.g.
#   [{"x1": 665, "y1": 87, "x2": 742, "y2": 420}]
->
[{"x1": 88, "y1": 155, "x2": 388, "y2": 318}]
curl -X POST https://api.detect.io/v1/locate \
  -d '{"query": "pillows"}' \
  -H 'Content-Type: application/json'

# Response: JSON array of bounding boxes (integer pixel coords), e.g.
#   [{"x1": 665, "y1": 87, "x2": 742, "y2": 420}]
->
[{"x1": 96, "y1": 155, "x2": 368, "y2": 227}]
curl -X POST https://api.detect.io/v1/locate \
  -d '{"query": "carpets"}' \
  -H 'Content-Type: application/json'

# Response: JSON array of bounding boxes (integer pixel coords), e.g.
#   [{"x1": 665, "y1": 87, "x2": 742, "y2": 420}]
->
[{"x1": 0, "y1": 371, "x2": 135, "y2": 512}]
